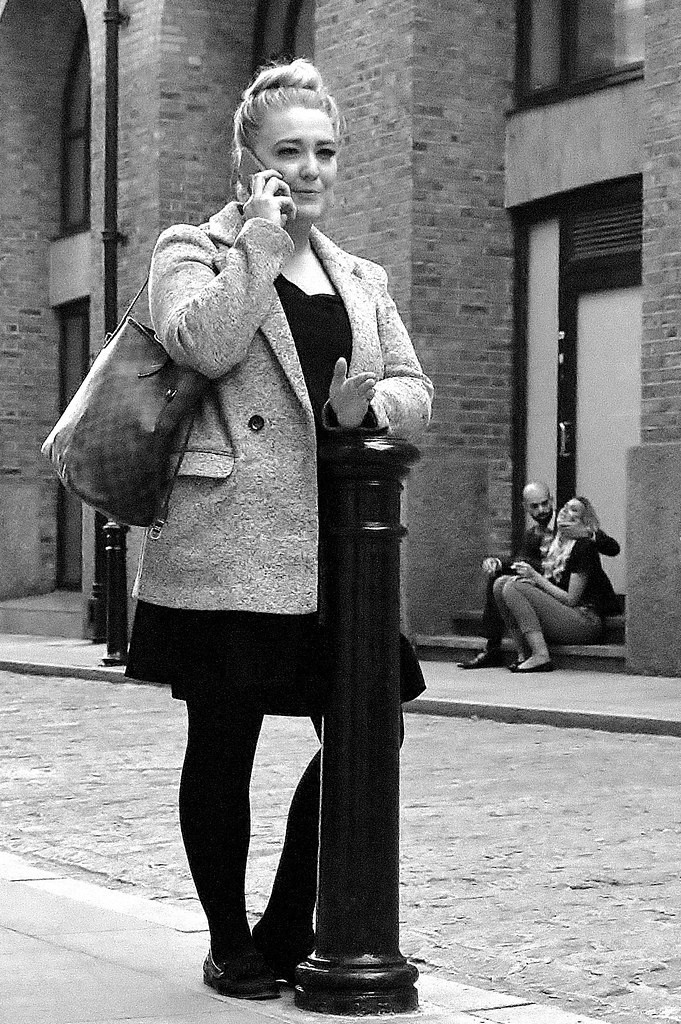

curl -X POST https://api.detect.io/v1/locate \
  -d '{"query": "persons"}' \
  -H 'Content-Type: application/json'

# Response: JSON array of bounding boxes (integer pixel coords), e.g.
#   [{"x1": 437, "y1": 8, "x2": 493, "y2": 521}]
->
[
  {"x1": 122, "y1": 59, "x2": 434, "y2": 1001},
  {"x1": 459, "y1": 481, "x2": 621, "y2": 673}
]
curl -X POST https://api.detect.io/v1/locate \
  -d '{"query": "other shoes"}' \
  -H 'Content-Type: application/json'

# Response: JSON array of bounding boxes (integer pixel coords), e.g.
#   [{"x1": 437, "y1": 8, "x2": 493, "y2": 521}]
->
[
  {"x1": 203, "y1": 948, "x2": 281, "y2": 999},
  {"x1": 251, "y1": 929, "x2": 316, "y2": 984},
  {"x1": 457, "y1": 650, "x2": 503, "y2": 669},
  {"x1": 508, "y1": 656, "x2": 532, "y2": 672},
  {"x1": 510, "y1": 660, "x2": 553, "y2": 673}
]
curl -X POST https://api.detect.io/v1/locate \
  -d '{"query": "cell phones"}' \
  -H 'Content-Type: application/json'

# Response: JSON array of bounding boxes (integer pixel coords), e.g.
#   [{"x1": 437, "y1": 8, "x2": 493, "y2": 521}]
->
[{"x1": 237, "y1": 147, "x2": 281, "y2": 196}]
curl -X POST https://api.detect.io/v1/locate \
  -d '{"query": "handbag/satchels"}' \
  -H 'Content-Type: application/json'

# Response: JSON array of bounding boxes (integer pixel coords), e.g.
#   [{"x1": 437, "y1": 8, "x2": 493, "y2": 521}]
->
[{"x1": 41, "y1": 273, "x2": 208, "y2": 527}]
